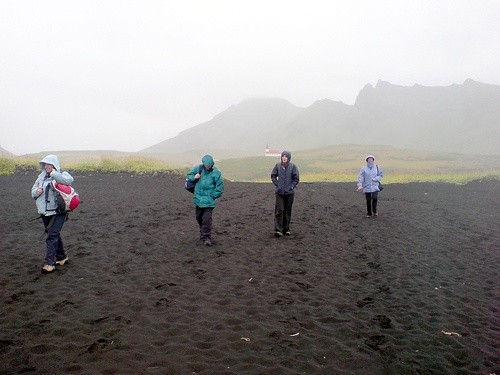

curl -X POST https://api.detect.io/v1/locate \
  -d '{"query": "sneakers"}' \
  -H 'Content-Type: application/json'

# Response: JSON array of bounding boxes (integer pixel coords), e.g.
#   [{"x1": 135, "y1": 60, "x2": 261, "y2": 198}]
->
[
  {"x1": 41, "y1": 264, "x2": 56, "y2": 273},
  {"x1": 56, "y1": 255, "x2": 69, "y2": 268}
]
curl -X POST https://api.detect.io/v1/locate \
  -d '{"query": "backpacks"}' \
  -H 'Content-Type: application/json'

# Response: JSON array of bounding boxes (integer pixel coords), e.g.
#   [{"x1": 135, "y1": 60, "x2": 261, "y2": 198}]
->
[
  {"x1": 44, "y1": 170, "x2": 81, "y2": 213},
  {"x1": 185, "y1": 164, "x2": 204, "y2": 193}
]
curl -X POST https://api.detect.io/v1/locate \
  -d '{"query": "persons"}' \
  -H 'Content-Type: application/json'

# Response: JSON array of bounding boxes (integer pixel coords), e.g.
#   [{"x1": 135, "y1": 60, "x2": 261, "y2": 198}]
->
[
  {"x1": 31, "y1": 154, "x2": 73, "y2": 274},
  {"x1": 356, "y1": 154, "x2": 382, "y2": 218},
  {"x1": 271, "y1": 150, "x2": 299, "y2": 237},
  {"x1": 186, "y1": 155, "x2": 223, "y2": 247}
]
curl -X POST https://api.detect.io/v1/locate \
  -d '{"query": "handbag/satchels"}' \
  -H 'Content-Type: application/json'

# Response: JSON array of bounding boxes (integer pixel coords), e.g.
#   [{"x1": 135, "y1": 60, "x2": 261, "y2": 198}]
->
[{"x1": 378, "y1": 182, "x2": 383, "y2": 191}]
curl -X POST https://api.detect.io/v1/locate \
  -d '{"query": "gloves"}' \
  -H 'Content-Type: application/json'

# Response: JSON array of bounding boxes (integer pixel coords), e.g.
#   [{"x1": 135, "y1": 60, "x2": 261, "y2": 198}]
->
[{"x1": 357, "y1": 185, "x2": 363, "y2": 192}]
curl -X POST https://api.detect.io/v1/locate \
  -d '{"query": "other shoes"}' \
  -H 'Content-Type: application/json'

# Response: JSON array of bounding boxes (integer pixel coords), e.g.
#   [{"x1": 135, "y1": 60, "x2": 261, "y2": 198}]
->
[
  {"x1": 204, "y1": 239, "x2": 211, "y2": 247},
  {"x1": 282, "y1": 230, "x2": 292, "y2": 236},
  {"x1": 274, "y1": 231, "x2": 283, "y2": 238},
  {"x1": 365, "y1": 211, "x2": 378, "y2": 218}
]
